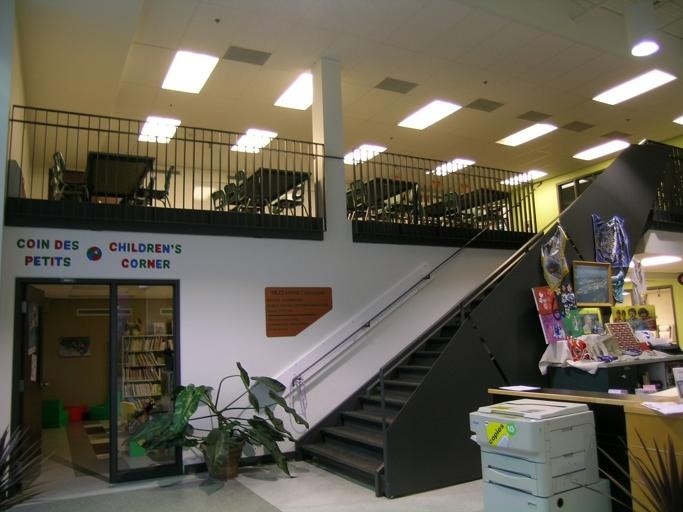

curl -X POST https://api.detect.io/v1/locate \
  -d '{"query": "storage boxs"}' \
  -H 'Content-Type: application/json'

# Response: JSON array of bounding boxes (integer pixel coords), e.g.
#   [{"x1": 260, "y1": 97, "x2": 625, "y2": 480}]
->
[{"x1": 469, "y1": 400, "x2": 612, "y2": 511}]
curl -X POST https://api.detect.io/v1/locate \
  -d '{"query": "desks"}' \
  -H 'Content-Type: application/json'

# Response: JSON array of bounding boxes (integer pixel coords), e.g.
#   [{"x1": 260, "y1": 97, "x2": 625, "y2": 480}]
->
[{"x1": 487, "y1": 383, "x2": 682, "y2": 511}]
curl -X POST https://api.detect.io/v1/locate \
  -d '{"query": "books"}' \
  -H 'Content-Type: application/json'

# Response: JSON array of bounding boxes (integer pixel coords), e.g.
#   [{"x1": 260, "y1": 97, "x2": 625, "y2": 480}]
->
[
  {"x1": 149, "y1": 322, "x2": 167, "y2": 335},
  {"x1": 124, "y1": 336, "x2": 175, "y2": 413}
]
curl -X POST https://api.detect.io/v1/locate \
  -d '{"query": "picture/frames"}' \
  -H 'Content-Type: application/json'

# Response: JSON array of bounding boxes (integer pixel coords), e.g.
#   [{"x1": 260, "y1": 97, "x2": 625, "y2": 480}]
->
[
  {"x1": 573, "y1": 260, "x2": 613, "y2": 308},
  {"x1": 607, "y1": 285, "x2": 678, "y2": 351}
]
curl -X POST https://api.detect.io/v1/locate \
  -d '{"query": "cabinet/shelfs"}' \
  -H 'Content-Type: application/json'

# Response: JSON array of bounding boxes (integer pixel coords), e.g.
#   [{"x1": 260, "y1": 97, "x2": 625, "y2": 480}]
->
[
  {"x1": 121, "y1": 333, "x2": 174, "y2": 412},
  {"x1": 542, "y1": 354, "x2": 683, "y2": 392}
]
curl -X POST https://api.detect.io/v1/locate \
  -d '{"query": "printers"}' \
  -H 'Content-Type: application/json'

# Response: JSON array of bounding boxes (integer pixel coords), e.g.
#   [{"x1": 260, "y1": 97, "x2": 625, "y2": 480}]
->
[{"x1": 467, "y1": 396, "x2": 601, "y2": 500}]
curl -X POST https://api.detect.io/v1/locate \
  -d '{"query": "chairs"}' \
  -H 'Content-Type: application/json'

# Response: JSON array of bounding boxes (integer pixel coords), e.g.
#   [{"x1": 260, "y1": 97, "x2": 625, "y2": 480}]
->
[
  {"x1": 48, "y1": 149, "x2": 176, "y2": 208},
  {"x1": 428, "y1": 186, "x2": 514, "y2": 231},
  {"x1": 208, "y1": 167, "x2": 312, "y2": 218},
  {"x1": 345, "y1": 176, "x2": 425, "y2": 226}
]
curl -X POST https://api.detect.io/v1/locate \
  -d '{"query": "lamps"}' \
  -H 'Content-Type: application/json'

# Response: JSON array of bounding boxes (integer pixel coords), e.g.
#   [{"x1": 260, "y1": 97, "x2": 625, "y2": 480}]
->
[{"x1": 623, "y1": 0, "x2": 659, "y2": 58}]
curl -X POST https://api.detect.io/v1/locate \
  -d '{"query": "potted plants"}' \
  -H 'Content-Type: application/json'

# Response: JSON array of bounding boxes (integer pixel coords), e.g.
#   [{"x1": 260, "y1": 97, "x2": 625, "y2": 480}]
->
[{"x1": 132, "y1": 361, "x2": 310, "y2": 482}]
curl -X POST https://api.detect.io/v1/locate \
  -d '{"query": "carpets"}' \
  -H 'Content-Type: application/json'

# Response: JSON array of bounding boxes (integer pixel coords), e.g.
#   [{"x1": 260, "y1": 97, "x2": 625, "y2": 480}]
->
[{"x1": 66, "y1": 420, "x2": 110, "y2": 478}]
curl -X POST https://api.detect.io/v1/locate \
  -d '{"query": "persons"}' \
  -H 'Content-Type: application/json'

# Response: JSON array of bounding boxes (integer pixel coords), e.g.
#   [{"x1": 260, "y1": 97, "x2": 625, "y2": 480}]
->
[{"x1": 613, "y1": 308, "x2": 656, "y2": 331}]
